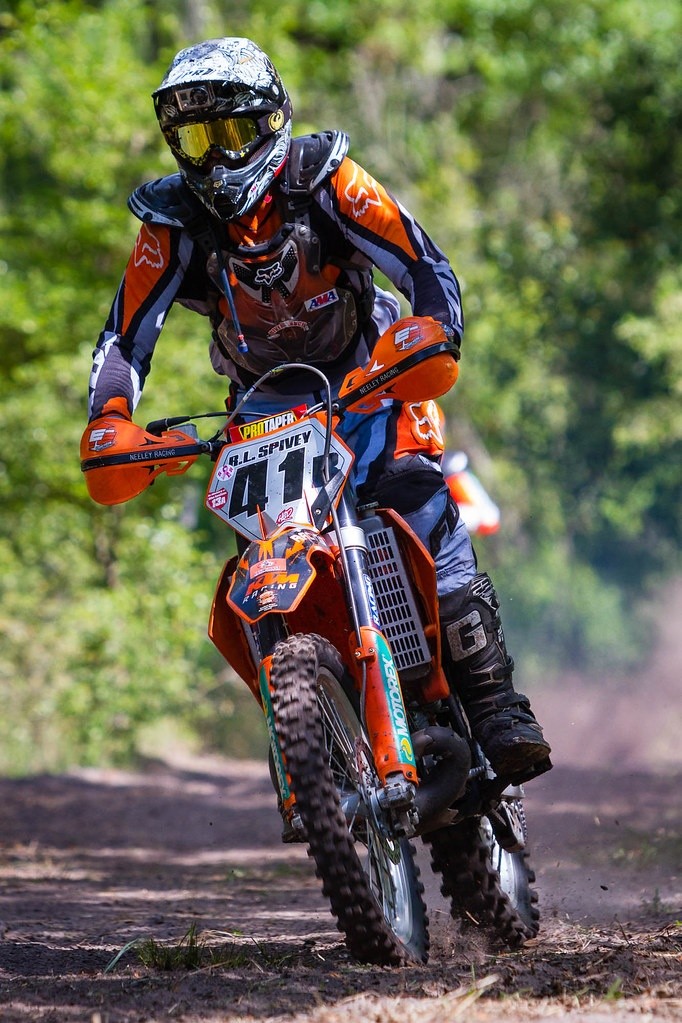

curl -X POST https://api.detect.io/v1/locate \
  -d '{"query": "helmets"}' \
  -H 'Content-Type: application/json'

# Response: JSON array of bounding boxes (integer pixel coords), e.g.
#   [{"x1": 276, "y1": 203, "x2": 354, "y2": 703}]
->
[{"x1": 150, "y1": 37, "x2": 292, "y2": 221}]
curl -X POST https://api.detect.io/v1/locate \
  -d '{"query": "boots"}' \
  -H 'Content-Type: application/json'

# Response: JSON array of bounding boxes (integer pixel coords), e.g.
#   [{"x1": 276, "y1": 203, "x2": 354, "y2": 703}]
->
[
  {"x1": 268, "y1": 740, "x2": 304, "y2": 845},
  {"x1": 442, "y1": 569, "x2": 552, "y2": 784}
]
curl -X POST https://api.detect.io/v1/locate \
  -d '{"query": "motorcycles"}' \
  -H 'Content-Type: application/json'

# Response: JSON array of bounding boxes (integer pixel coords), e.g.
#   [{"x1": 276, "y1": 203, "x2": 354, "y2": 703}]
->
[{"x1": 74, "y1": 313, "x2": 553, "y2": 970}]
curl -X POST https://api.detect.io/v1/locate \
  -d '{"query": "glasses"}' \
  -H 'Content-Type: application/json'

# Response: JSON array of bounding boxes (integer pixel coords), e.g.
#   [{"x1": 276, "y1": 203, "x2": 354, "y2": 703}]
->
[{"x1": 165, "y1": 116, "x2": 261, "y2": 165}]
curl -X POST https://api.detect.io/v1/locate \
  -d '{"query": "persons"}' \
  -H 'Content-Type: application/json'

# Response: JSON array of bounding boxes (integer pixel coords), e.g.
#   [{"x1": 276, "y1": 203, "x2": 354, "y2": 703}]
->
[{"x1": 78, "y1": 37, "x2": 554, "y2": 842}]
